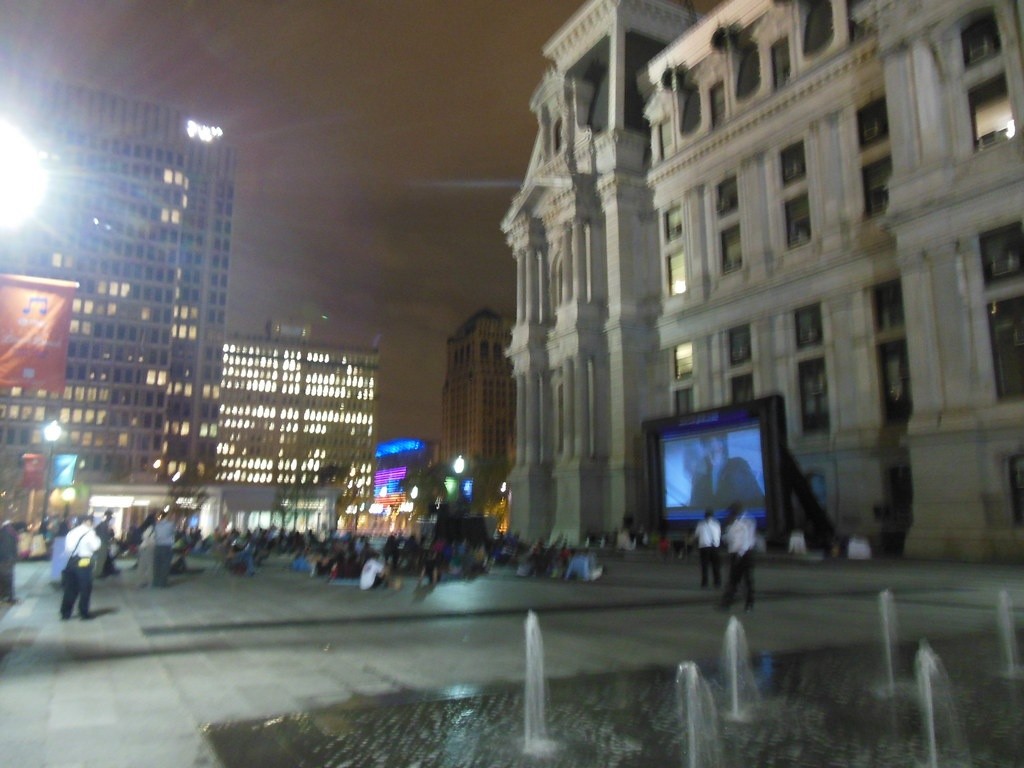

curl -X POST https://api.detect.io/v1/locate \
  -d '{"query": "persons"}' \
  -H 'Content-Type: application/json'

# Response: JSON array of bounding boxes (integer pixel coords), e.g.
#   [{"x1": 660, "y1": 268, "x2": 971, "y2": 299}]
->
[
  {"x1": 61, "y1": 515, "x2": 102, "y2": 620},
  {"x1": 685, "y1": 431, "x2": 765, "y2": 509},
  {"x1": 0, "y1": 507, "x2": 673, "y2": 604},
  {"x1": 749, "y1": 526, "x2": 767, "y2": 556},
  {"x1": 688, "y1": 507, "x2": 722, "y2": 589},
  {"x1": 714, "y1": 503, "x2": 758, "y2": 614}
]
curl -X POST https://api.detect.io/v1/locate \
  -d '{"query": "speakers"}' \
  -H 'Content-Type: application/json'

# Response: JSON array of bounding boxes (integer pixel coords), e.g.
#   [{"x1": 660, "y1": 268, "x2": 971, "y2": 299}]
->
[
  {"x1": 874, "y1": 505, "x2": 890, "y2": 521},
  {"x1": 623, "y1": 517, "x2": 635, "y2": 529}
]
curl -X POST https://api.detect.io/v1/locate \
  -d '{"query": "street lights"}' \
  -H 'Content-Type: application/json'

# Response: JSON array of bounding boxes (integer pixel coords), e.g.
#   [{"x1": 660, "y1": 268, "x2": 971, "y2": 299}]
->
[
  {"x1": 62, "y1": 486, "x2": 77, "y2": 532},
  {"x1": 38, "y1": 420, "x2": 63, "y2": 536},
  {"x1": 453, "y1": 453, "x2": 468, "y2": 505}
]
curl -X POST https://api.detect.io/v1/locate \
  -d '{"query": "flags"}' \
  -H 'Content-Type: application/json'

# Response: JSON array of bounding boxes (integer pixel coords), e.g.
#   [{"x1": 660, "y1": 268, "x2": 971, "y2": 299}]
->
[
  {"x1": 0, "y1": 273, "x2": 77, "y2": 397},
  {"x1": 22, "y1": 453, "x2": 48, "y2": 490},
  {"x1": 50, "y1": 454, "x2": 79, "y2": 487}
]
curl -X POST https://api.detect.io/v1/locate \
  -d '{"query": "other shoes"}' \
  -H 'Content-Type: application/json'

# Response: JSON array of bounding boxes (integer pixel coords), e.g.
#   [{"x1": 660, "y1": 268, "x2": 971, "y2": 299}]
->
[
  {"x1": 744, "y1": 603, "x2": 752, "y2": 614},
  {"x1": 712, "y1": 603, "x2": 728, "y2": 611}
]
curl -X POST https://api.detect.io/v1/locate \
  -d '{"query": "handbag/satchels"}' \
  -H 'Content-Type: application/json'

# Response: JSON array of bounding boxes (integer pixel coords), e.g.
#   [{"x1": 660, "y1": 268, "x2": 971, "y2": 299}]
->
[{"x1": 57, "y1": 552, "x2": 72, "y2": 571}]
